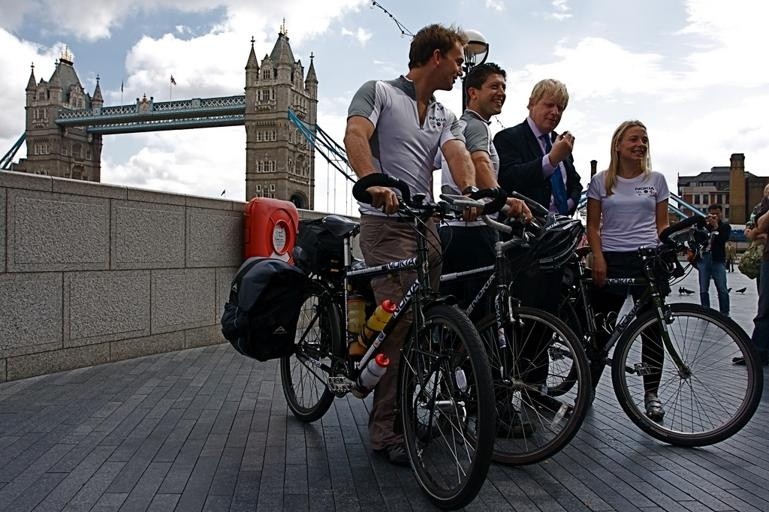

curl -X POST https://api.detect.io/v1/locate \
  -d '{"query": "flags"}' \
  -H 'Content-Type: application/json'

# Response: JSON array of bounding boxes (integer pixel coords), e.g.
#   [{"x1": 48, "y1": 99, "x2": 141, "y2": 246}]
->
[
  {"x1": 171, "y1": 75, "x2": 177, "y2": 86},
  {"x1": 121, "y1": 82, "x2": 124, "y2": 93}
]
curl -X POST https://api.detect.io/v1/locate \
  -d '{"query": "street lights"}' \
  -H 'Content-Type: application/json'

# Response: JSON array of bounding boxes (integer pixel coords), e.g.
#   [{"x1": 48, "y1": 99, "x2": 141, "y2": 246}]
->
[{"x1": 456, "y1": 28, "x2": 492, "y2": 116}]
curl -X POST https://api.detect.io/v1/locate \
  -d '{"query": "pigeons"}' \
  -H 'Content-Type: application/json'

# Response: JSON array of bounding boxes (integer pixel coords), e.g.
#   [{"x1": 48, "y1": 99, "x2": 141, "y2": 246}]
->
[
  {"x1": 684, "y1": 289, "x2": 695, "y2": 294},
  {"x1": 682, "y1": 287, "x2": 685, "y2": 294},
  {"x1": 727, "y1": 288, "x2": 732, "y2": 293},
  {"x1": 736, "y1": 287, "x2": 747, "y2": 295},
  {"x1": 679, "y1": 286, "x2": 683, "y2": 293}
]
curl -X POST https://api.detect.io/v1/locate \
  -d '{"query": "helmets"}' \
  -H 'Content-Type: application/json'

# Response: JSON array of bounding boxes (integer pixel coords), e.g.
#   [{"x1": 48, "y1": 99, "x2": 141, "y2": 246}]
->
[{"x1": 535, "y1": 214, "x2": 584, "y2": 273}]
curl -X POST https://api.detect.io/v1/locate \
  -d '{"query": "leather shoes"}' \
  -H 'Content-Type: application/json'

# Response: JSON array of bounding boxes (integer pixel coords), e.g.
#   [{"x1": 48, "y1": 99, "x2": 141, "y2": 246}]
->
[{"x1": 733, "y1": 357, "x2": 768, "y2": 365}]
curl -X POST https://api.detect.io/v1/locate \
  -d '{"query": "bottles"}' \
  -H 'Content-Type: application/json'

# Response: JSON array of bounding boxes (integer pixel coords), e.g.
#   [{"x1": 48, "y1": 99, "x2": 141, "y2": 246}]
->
[
  {"x1": 347, "y1": 295, "x2": 365, "y2": 355},
  {"x1": 358, "y1": 298, "x2": 399, "y2": 350},
  {"x1": 349, "y1": 352, "x2": 390, "y2": 400}
]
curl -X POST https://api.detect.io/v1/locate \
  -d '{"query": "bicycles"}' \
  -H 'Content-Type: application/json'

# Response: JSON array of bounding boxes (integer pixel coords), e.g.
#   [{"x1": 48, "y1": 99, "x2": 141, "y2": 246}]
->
[
  {"x1": 385, "y1": 184, "x2": 594, "y2": 467},
  {"x1": 513, "y1": 206, "x2": 766, "y2": 449},
  {"x1": 275, "y1": 175, "x2": 512, "y2": 507}
]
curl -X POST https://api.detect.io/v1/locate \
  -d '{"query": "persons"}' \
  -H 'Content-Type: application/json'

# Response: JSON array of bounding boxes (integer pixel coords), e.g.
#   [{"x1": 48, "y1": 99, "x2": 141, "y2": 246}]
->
[
  {"x1": 343, "y1": 24, "x2": 484, "y2": 469},
  {"x1": 687, "y1": 183, "x2": 769, "y2": 368},
  {"x1": 575, "y1": 119, "x2": 670, "y2": 422},
  {"x1": 431, "y1": 61, "x2": 536, "y2": 437},
  {"x1": 492, "y1": 79, "x2": 582, "y2": 411}
]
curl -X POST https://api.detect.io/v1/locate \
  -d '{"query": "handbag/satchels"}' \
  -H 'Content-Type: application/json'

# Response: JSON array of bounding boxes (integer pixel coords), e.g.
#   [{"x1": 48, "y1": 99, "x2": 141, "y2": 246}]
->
[
  {"x1": 739, "y1": 243, "x2": 764, "y2": 279},
  {"x1": 220, "y1": 256, "x2": 312, "y2": 361},
  {"x1": 298, "y1": 216, "x2": 354, "y2": 278}
]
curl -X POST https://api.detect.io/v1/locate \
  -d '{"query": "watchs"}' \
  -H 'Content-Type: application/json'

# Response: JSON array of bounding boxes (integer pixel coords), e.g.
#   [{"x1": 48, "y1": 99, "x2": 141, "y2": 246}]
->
[{"x1": 461, "y1": 186, "x2": 480, "y2": 195}]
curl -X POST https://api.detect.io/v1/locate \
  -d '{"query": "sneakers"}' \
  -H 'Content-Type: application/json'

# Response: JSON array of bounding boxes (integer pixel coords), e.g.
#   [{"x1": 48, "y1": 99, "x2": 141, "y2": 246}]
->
[
  {"x1": 643, "y1": 393, "x2": 664, "y2": 421},
  {"x1": 496, "y1": 403, "x2": 536, "y2": 434},
  {"x1": 373, "y1": 415, "x2": 442, "y2": 466}
]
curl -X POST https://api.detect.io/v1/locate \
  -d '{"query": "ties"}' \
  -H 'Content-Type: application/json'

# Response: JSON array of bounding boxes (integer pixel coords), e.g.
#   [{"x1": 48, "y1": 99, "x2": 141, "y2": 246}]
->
[{"x1": 542, "y1": 134, "x2": 568, "y2": 216}]
